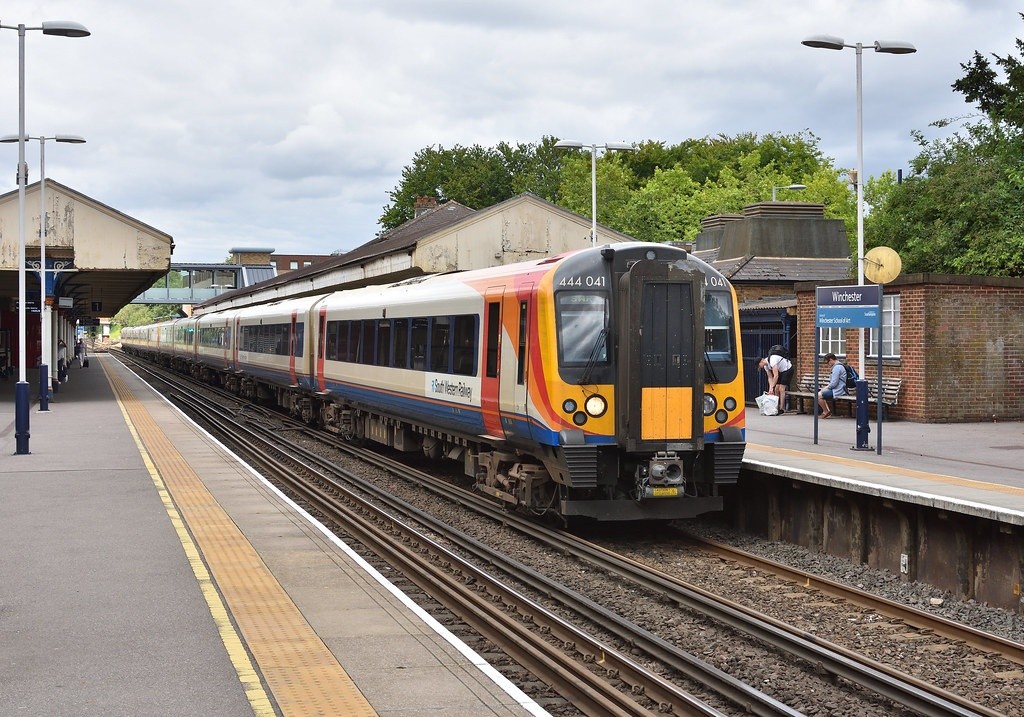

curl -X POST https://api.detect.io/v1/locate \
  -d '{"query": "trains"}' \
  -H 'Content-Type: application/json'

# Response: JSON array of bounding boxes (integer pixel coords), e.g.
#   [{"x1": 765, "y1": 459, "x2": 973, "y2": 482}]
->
[{"x1": 119, "y1": 241, "x2": 748, "y2": 529}]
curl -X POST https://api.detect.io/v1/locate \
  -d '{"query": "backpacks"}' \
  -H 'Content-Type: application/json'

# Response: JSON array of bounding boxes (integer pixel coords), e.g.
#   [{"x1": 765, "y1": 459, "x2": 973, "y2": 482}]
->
[
  {"x1": 831, "y1": 363, "x2": 858, "y2": 388},
  {"x1": 767, "y1": 344, "x2": 792, "y2": 364}
]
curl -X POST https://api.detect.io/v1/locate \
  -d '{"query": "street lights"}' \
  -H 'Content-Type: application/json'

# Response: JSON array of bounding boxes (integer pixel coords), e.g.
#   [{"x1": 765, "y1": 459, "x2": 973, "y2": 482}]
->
[
  {"x1": 0, "y1": 129, "x2": 86, "y2": 411},
  {"x1": 772, "y1": 183, "x2": 807, "y2": 201},
  {"x1": 798, "y1": 33, "x2": 918, "y2": 450},
  {"x1": 1, "y1": 19, "x2": 92, "y2": 456},
  {"x1": 553, "y1": 139, "x2": 635, "y2": 247}
]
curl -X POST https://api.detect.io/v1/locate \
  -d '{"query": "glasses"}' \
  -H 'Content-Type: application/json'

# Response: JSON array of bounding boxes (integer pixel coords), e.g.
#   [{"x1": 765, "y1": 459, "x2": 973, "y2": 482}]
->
[{"x1": 825, "y1": 360, "x2": 830, "y2": 364}]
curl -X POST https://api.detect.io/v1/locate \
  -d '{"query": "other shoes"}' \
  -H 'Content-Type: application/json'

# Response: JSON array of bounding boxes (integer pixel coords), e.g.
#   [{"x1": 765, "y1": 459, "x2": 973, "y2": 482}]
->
[
  {"x1": 818, "y1": 410, "x2": 831, "y2": 419},
  {"x1": 779, "y1": 409, "x2": 785, "y2": 414}
]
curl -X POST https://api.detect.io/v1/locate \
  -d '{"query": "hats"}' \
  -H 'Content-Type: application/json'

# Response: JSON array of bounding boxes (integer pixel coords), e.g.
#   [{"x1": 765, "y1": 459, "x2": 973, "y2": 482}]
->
[{"x1": 754, "y1": 357, "x2": 762, "y2": 369}]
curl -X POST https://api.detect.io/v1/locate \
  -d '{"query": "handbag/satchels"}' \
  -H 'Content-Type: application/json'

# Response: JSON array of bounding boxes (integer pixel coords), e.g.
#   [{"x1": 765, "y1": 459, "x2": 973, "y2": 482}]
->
[{"x1": 755, "y1": 391, "x2": 779, "y2": 416}]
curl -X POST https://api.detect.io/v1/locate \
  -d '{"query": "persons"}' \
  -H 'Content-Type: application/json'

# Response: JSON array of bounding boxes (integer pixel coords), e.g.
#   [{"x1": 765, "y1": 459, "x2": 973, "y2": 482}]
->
[
  {"x1": 818, "y1": 353, "x2": 849, "y2": 419},
  {"x1": 753, "y1": 355, "x2": 794, "y2": 414},
  {"x1": 78, "y1": 338, "x2": 87, "y2": 369}
]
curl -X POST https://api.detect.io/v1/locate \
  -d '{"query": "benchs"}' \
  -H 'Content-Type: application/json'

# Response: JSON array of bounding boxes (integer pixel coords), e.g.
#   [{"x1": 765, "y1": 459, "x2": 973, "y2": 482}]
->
[{"x1": 788, "y1": 376, "x2": 903, "y2": 421}]
